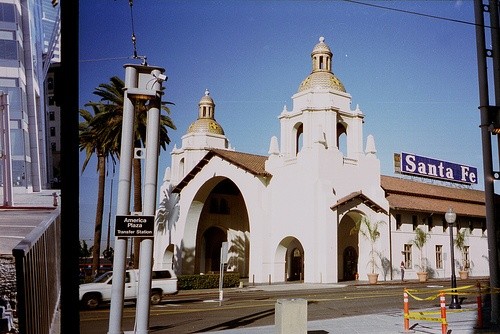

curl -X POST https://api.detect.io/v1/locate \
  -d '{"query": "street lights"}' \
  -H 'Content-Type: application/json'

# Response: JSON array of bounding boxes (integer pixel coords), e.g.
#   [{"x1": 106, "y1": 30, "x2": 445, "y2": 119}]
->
[{"x1": 445, "y1": 206, "x2": 462, "y2": 309}]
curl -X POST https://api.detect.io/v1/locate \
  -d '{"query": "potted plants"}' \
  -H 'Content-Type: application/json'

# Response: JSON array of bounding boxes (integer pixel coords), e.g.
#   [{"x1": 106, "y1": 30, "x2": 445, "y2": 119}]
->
[
  {"x1": 414, "y1": 227, "x2": 428, "y2": 281},
  {"x1": 454, "y1": 231, "x2": 468, "y2": 280},
  {"x1": 349, "y1": 216, "x2": 387, "y2": 284}
]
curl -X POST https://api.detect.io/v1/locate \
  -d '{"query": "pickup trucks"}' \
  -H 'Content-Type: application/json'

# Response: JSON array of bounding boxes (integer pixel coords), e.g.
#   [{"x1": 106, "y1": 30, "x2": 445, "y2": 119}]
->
[{"x1": 78, "y1": 269, "x2": 178, "y2": 310}]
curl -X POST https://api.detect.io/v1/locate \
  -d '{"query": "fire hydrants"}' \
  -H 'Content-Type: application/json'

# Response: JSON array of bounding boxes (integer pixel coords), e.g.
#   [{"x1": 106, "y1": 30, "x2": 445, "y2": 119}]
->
[{"x1": 52, "y1": 192, "x2": 59, "y2": 205}]
